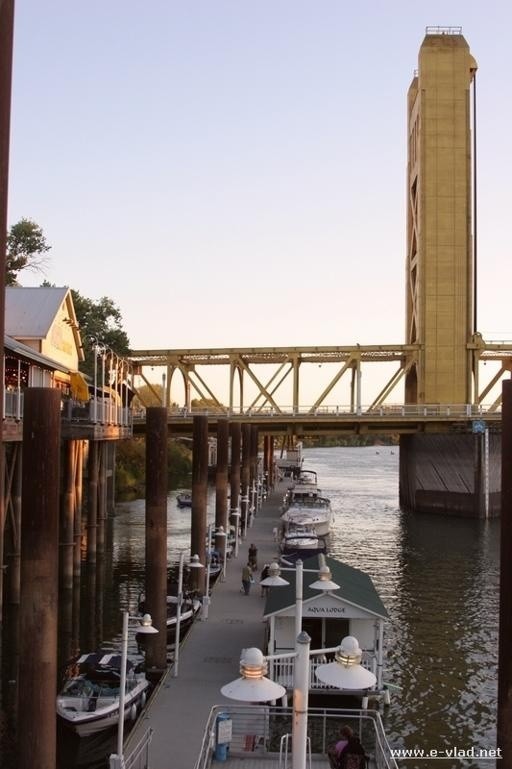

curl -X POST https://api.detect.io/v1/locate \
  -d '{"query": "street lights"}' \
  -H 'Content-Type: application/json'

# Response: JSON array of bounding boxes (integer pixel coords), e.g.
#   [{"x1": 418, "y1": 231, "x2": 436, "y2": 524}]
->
[
  {"x1": 173, "y1": 551, "x2": 204, "y2": 677},
  {"x1": 204, "y1": 524, "x2": 227, "y2": 620},
  {"x1": 222, "y1": 470, "x2": 269, "y2": 577},
  {"x1": 220, "y1": 631, "x2": 377, "y2": 768},
  {"x1": 117, "y1": 610, "x2": 159, "y2": 757},
  {"x1": 258, "y1": 558, "x2": 341, "y2": 644}
]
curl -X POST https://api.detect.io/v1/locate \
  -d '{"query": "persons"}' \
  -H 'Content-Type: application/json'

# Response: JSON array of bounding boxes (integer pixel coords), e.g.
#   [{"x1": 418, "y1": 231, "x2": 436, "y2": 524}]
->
[
  {"x1": 63, "y1": 662, "x2": 121, "y2": 696},
  {"x1": 259, "y1": 563, "x2": 270, "y2": 598},
  {"x1": 240, "y1": 561, "x2": 254, "y2": 597},
  {"x1": 329, "y1": 725, "x2": 355, "y2": 769},
  {"x1": 338, "y1": 734, "x2": 368, "y2": 769},
  {"x1": 247, "y1": 542, "x2": 259, "y2": 573}
]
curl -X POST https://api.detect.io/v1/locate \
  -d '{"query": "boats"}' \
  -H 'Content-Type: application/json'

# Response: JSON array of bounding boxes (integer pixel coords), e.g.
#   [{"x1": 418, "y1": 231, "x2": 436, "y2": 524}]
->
[
  {"x1": 56, "y1": 649, "x2": 151, "y2": 740},
  {"x1": 176, "y1": 490, "x2": 192, "y2": 509},
  {"x1": 279, "y1": 470, "x2": 331, "y2": 553},
  {"x1": 138, "y1": 592, "x2": 201, "y2": 644}
]
